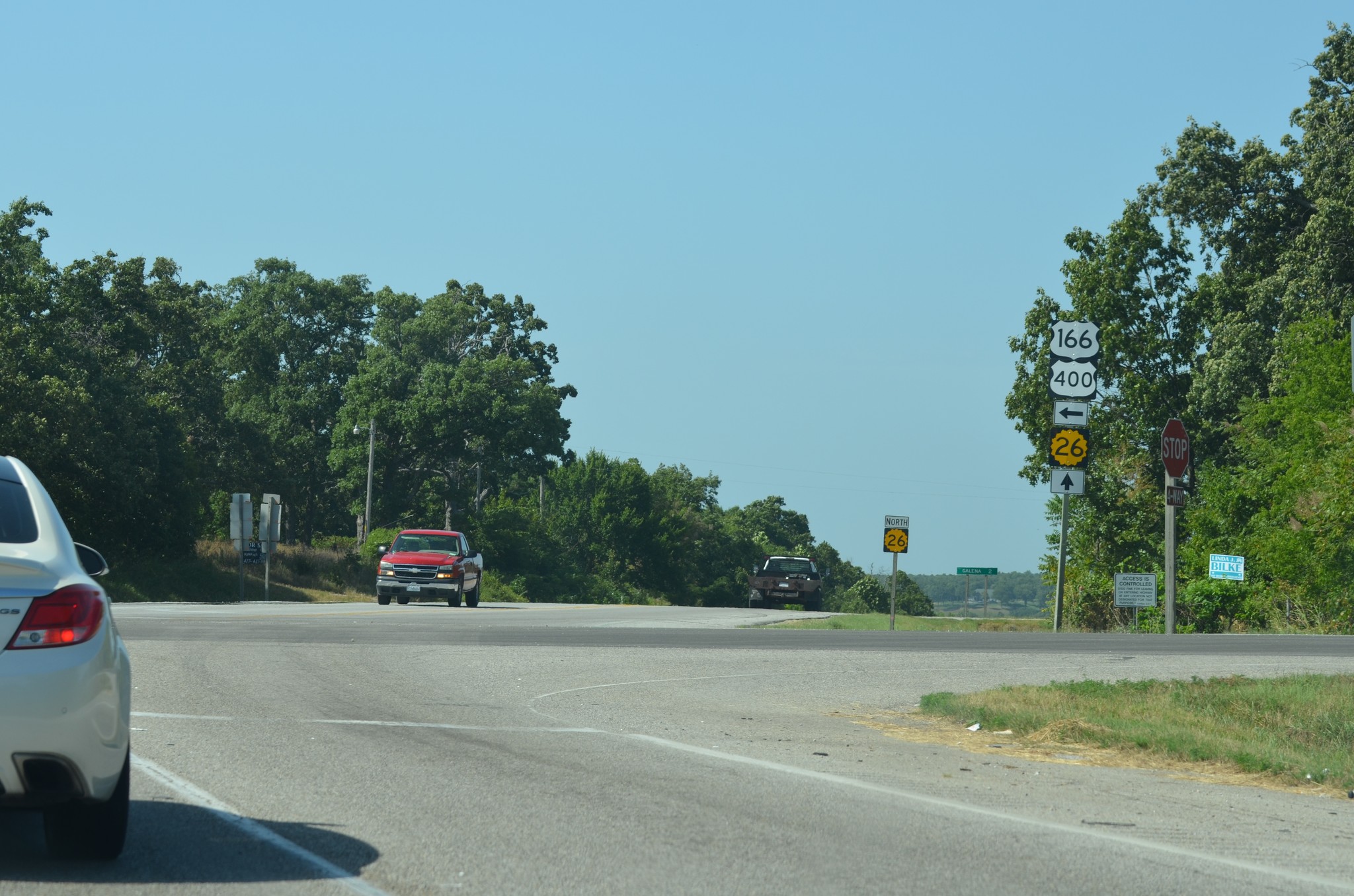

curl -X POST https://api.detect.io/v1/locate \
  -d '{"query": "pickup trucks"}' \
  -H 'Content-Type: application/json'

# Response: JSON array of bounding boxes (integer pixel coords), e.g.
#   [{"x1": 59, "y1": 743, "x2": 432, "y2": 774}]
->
[{"x1": 375, "y1": 530, "x2": 484, "y2": 606}]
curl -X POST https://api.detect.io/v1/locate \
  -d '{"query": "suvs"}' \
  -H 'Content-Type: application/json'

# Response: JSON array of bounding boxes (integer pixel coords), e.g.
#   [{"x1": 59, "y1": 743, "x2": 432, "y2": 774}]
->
[{"x1": 748, "y1": 555, "x2": 824, "y2": 611}]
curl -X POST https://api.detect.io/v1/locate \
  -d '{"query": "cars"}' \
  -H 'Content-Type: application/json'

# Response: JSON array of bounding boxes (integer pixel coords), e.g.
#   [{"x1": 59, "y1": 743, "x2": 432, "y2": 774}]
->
[{"x1": 0, "y1": 455, "x2": 131, "y2": 857}]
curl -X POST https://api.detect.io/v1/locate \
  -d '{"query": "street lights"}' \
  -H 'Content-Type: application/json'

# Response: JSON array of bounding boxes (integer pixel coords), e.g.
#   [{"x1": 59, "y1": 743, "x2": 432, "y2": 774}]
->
[{"x1": 353, "y1": 419, "x2": 375, "y2": 533}]
